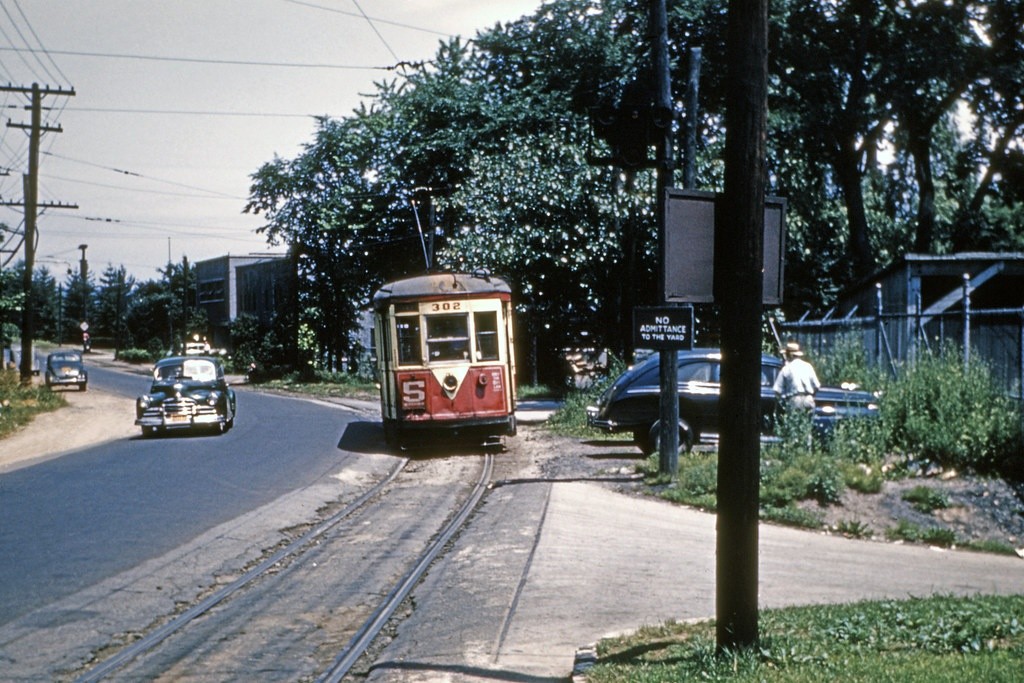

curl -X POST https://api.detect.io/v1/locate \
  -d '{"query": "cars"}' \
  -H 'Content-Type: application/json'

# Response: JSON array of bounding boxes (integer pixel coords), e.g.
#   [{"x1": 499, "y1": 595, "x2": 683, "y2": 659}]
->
[
  {"x1": 136, "y1": 361, "x2": 236, "y2": 436},
  {"x1": 10, "y1": 347, "x2": 42, "y2": 374},
  {"x1": 45, "y1": 349, "x2": 89, "y2": 392},
  {"x1": 168, "y1": 343, "x2": 208, "y2": 355},
  {"x1": 586, "y1": 346, "x2": 880, "y2": 455}
]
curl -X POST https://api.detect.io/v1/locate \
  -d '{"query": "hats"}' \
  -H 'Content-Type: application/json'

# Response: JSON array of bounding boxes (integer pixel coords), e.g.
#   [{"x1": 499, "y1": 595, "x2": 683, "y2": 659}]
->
[{"x1": 779, "y1": 343, "x2": 804, "y2": 357}]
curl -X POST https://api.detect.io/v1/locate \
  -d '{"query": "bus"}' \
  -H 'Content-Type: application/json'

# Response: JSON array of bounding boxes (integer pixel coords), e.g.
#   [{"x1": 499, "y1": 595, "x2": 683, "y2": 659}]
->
[{"x1": 371, "y1": 270, "x2": 517, "y2": 446}]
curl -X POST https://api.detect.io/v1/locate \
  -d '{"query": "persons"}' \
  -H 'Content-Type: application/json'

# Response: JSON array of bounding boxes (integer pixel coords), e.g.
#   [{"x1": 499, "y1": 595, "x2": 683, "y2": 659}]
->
[{"x1": 772, "y1": 342, "x2": 821, "y2": 452}]
